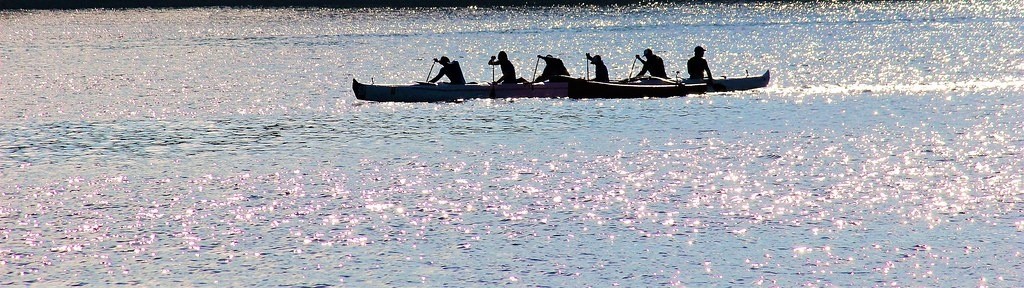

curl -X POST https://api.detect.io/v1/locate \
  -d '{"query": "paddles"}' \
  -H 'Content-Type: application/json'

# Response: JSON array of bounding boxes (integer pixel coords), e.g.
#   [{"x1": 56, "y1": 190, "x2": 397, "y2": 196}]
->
[
  {"x1": 426, "y1": 61, "x2": 436, "y2": 81},
  {"x1": 533, "y1": 57, "x2": 539, "y2": 81},
  {"x1": 492, "y1": 60, "x2": 495, "y2": 81},
  {"x1": 628, "y1": 57, "x2": 638, "y2": 79},
  {"x1": 586, "y1": 53, "x2": 590, "y2": 80}
]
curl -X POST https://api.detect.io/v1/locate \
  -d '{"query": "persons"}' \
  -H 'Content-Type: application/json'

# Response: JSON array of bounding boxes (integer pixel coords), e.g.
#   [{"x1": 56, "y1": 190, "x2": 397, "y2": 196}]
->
[
  {"x1": 429, "y1": 56, "x2": 466, "y2": 84},
  {"x1": 586, "y1": 54, "x2": 609, "y2": 82},
  {"x1": 633, "y1": 48, "x2": 667, "y2": 79},
  {"x1": 532, "y1": 55, "x2": 570, "y2": 84},
  {"x1": 687, "y1": 46, "x2": 712, "y2": 79},
  {"x1": 488, "y1": 51, "x2": 517, "y2": 85}
]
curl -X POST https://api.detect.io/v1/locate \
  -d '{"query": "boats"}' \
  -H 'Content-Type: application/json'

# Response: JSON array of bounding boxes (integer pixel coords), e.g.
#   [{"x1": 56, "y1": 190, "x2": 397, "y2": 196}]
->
[
  {"x1": 555, "y1": 74, "x2": 707, "y2": 100},
  {"x1": 351, "y1": 70, "x2": 771, "y2": 104}
]
